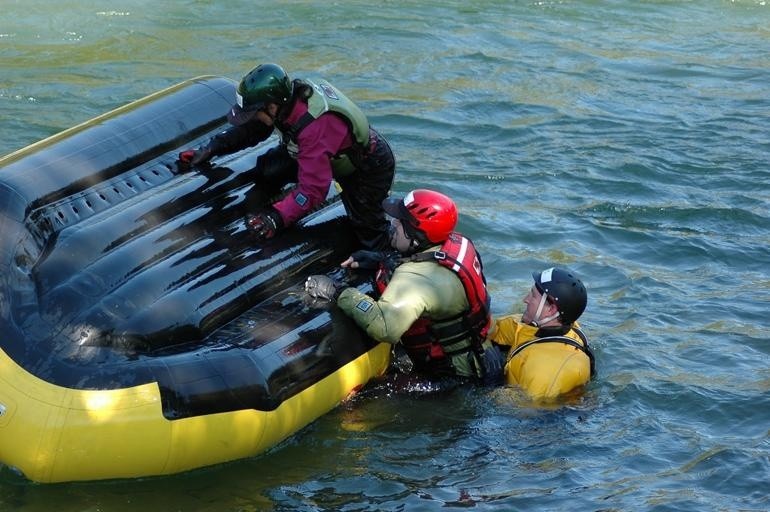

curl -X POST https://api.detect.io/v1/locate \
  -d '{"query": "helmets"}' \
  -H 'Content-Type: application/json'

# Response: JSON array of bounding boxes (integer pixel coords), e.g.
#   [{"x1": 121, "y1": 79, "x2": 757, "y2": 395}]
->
[
  {"x1": 382, "y1": 186, "x2": 460, "y2": 247},
  {"x1": 531, "y1": 265, "x2": 586, "y2": 326},
  {"x1": 227, "y1": 60, "x2": 290, "y2": 125}
]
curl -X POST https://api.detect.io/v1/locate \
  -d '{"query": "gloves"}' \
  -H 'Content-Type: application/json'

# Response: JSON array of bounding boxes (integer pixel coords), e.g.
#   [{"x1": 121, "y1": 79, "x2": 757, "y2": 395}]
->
[
  {"x1": 306, "y1": 274, "x2": 336, "y2": 300},
  {"x1": 178, "y1": 144, "x2": 213, "y2": 168},
  {"x1": 350, "y1": 247, "x2": 380, "y2": 271},
  {"x1": 244, "y1": 211, "x2": 282, "y2": 245}
]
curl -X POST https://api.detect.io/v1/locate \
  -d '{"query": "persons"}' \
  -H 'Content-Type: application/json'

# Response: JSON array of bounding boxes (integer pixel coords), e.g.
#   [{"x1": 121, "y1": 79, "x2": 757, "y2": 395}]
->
[
  {"x1": 487, "y1": 266, "x2": 596, "y2": 401},
  {"x1": 301, "y1": 187, "x2": 506, "y2": 393},
  {"x1": 177, "y1": 62, "x2": 397, "y2": 247}
]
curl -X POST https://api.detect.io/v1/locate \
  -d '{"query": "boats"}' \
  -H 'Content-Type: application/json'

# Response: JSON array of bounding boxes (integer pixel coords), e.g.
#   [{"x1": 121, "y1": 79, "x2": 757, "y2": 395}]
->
[{"x1": 1, "y1": 74, "x2": 449, "y2": 473}]
